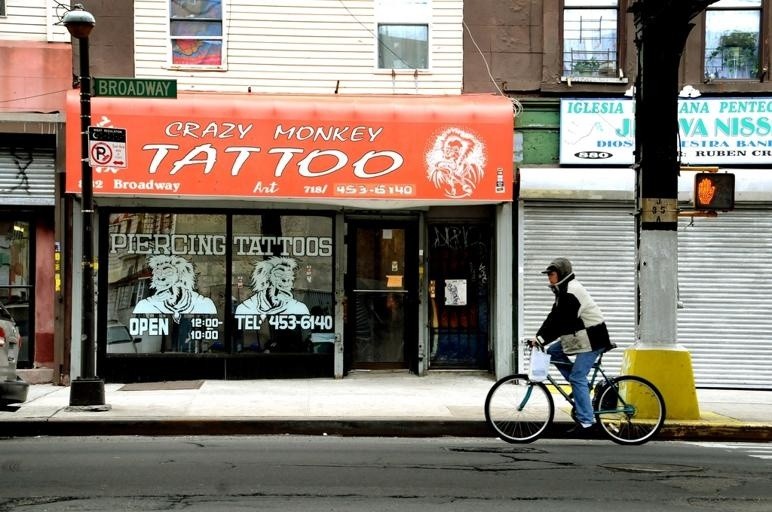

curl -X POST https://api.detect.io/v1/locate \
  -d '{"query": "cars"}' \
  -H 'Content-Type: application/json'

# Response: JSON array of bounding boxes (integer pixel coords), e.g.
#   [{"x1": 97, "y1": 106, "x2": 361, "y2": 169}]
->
[{"x1": 1, "y1": 299, "x2": 27, "y2": 411}]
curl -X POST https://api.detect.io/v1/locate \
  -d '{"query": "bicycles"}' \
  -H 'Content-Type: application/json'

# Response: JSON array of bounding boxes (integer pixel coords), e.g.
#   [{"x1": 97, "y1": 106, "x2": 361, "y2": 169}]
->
[{"x1": 486, "y1": 338, "x2": 666, "y2": 446}]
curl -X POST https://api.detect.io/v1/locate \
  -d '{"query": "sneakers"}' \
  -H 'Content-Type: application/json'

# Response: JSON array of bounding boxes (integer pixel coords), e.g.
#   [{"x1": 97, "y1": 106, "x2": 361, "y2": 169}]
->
[
  {"x1": 566, "y1": 423, "x2": 593, "y2": 434},
  {"x1": 566, "y1": 382, "x2": 593, "y2": 399}
]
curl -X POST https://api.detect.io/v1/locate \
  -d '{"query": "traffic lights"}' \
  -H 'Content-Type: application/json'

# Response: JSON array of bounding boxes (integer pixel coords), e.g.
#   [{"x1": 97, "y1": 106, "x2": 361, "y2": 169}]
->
[{"x1": 697, "y1": 170, "x2": 735, "y2": 211}]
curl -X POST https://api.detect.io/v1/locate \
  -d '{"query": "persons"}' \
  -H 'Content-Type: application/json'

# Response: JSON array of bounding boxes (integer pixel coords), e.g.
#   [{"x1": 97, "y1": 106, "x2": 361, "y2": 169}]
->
[{"x1": 532, "y1": 257, "x2": 611, "y2": 435}]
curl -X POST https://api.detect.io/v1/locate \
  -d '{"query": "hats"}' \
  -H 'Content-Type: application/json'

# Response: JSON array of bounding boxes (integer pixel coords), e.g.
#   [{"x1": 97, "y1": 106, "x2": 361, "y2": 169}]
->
[{"x1": 541, "y1": 265, "x2": 558, "y2": 274}]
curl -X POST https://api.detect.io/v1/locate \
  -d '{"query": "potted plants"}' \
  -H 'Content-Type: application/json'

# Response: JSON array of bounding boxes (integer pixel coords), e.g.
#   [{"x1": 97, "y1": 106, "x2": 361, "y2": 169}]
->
[
  {"x1": 707, "y1": 32, "x2": 760, "y2": 76},
  {"x1": 573, "y1": 56, "x2": 600, "y2": 80}
]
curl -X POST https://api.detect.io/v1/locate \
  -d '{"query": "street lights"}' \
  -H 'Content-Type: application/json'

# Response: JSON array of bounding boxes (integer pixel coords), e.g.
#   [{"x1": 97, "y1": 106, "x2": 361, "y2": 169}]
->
[{"x1": 61, "y1": 5, "x2": 111, "y2": 411}]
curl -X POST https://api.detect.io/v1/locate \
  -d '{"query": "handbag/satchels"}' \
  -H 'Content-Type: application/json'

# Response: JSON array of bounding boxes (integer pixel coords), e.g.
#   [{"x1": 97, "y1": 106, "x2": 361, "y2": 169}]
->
[{"x1": 527, "y1": 344, "x2": 551, "y2": 383}]
